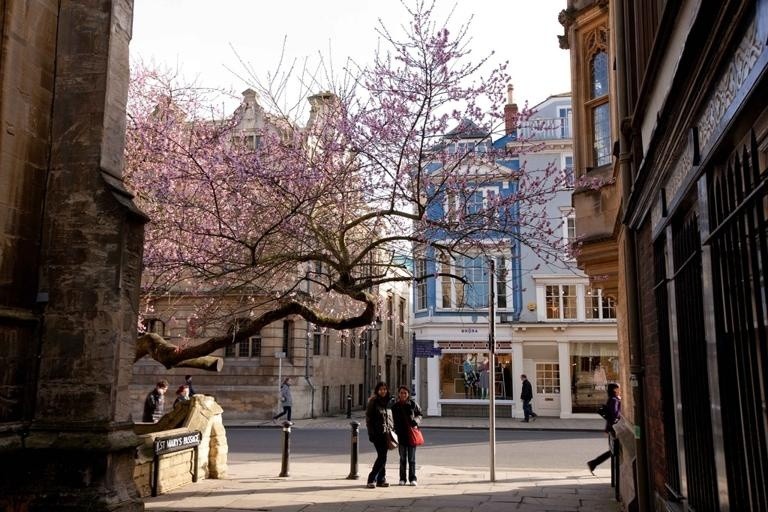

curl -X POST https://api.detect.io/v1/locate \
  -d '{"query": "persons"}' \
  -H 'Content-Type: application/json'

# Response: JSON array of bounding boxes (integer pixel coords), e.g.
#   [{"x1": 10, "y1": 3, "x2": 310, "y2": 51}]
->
[
  {"x1": 479, "y1": 353, "x2": 490, "y2": 399},
  {"x1": 520, "y1": 374, "x2": 538, "y2": 423},
  {"x1": 142, "y1": 379, "x2": 168, "y2": 422},
  {"x1": 463, "y1": 353, "x2": 477, "y2": 399},
  {"x1": 184, "y1": 375, "x2": 196, "y2": 398},
  {"x1": 586, "y1": 383, "x2": 624, "y2": 476},
  {"x1": 391, "y1": 385, "x2": 423, "y2": 486},
  {"x1": 173, "y1": 384, "x2": 190, "y2": 410},
  {"x1": 365, "y1": 382, "x2": 394, "y2": 488},
  {"x1": 272, "y1": 377, "x2": 296, "y2": 425}
]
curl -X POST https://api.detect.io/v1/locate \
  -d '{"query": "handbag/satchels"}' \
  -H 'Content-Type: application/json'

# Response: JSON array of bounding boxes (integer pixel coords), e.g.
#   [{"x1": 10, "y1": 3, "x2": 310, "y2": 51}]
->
[
  {"x1": 386, "y1": 430, "x2": 400, "y2": 449},
  {"x1": 599, "y1": 405, "x2": 612, "y2": 420},
  {"x1": 407, "y1": 427, "x2": 424, "y2": 446}
]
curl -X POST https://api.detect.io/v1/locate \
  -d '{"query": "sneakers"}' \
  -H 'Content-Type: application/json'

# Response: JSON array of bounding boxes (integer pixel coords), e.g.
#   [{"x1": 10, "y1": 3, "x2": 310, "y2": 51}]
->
[
  {"x1": 587, "y1": 460, "x2": 597, "y2": 476},
  {"x1": 398, "y1": 479, "x2": 417, "y2": 486},
  {"x1": 367, "y1": 481, "x2": 390, "y2": 487}
]
